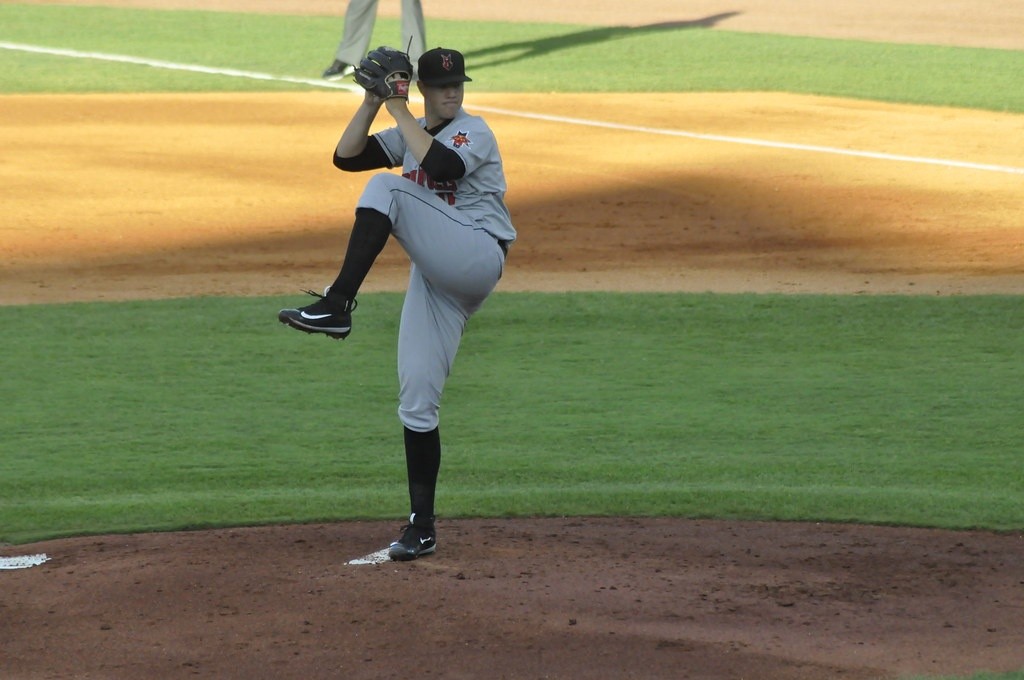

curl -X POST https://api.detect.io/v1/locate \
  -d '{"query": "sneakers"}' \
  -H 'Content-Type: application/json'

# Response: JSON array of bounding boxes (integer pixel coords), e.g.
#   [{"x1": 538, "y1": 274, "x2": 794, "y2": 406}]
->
[
  {"x1": 278, "y1": 288, "x2": 357, "y2": 341},
  {"x1": 389, "y1": 513, "x2": 436, "y2": 561},
  {"x1": 322, "y1": 59, "x2": 360, "y2": 80}
]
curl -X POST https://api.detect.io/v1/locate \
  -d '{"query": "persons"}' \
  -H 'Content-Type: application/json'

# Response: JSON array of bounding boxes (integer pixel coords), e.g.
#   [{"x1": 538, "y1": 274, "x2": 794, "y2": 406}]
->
[
  {"x1": 278, "y1": 45, "x2": 517, "y2": 562},
  {"x1": 322, "y1": 0, "x2": 426, "y2": 82}
]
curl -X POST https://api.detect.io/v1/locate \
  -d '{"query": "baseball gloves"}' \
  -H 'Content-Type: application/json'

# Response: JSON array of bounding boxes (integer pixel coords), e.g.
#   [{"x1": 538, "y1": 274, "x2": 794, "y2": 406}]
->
[{"x1": 355, "y1": 45, "x2": 414, "y2": 100}]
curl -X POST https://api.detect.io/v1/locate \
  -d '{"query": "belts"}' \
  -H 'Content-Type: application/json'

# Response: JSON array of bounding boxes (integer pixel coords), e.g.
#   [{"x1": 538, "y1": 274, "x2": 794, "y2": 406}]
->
[{"x1": 498, "y1": 239, "x2": 508, "y2": 259}]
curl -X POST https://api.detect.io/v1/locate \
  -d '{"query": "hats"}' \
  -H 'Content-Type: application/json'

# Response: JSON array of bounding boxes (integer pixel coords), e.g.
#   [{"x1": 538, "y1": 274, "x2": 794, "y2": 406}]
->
[{"x1": 417, "y1": 47, "x2": 472, "y2": 86}]
